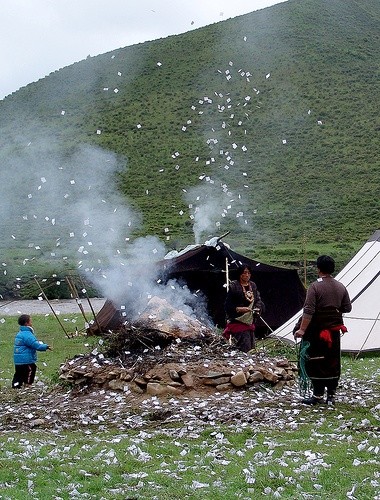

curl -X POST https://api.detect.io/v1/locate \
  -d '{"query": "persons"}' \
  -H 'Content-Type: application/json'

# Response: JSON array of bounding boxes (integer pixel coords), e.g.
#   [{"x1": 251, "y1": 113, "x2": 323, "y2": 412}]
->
[
  {"x1": 292, "y1": 255, "x2": 352, "y2": 405},
  {"x1": 10, "y1": 314, "x2": 51, "y2": 389},
  {"x1": 222, "y1": 264, "x2": 264, "y2": 354}
]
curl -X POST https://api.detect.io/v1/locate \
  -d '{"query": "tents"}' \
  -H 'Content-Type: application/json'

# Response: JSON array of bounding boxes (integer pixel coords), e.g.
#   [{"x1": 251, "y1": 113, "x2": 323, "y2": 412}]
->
[
  {"x1": 86, "y1": 228, "x2": 309, "y2": 343},
  {"x1": 261, "y1": 228, "x2": 380, "y2": 357}
]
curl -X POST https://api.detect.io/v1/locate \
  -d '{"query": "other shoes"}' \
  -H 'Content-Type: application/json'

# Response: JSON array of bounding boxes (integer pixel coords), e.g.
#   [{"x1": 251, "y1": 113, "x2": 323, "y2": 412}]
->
[{"x1": 301, "y1": 395, "x2": 335, "y2": 404}]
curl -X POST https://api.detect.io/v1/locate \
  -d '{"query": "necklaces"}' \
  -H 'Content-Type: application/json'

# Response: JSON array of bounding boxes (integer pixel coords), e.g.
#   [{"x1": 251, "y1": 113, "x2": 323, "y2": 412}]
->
[{"x1": 239, "y1": 279, "x2": 253, "y2": 301}]
